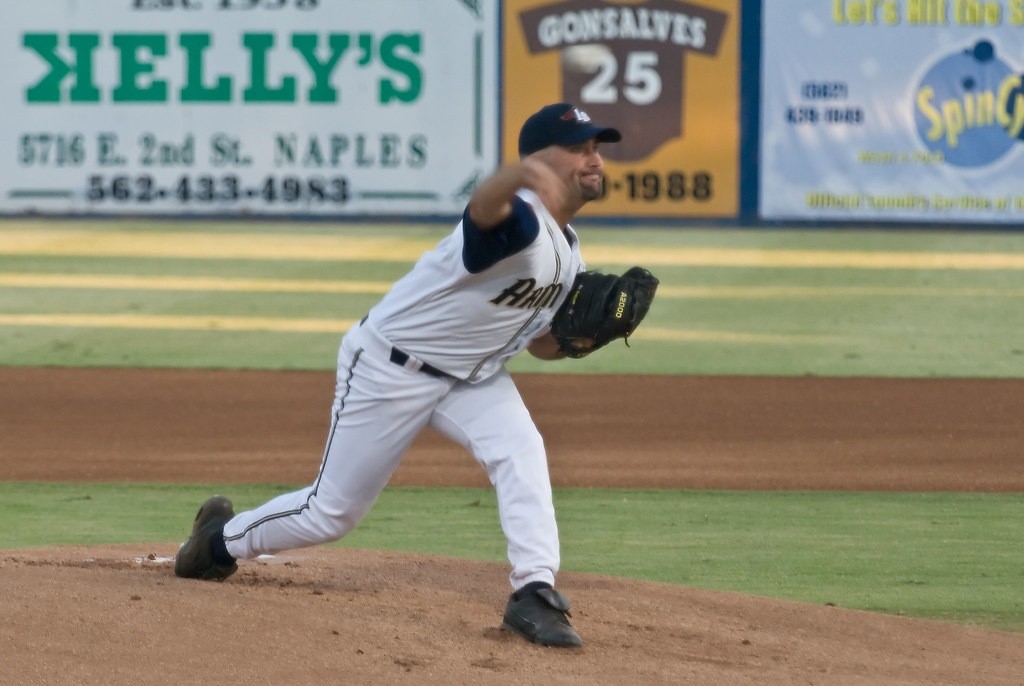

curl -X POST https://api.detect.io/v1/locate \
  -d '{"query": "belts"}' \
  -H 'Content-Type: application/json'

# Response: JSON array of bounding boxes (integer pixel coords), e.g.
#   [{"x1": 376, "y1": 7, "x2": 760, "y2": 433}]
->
[{"x1": 359, "y1": 314, "x2": 450, "y2": 377}]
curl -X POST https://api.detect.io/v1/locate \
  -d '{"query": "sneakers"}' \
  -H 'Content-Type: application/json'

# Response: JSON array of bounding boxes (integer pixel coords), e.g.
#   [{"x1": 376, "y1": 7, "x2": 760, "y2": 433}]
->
[
  {"x1": 501, "y1": 589, "x2": 583, "y2": 654},
  {"x1": 174, "y1": 497, "x2": 238, "y2": 582}
]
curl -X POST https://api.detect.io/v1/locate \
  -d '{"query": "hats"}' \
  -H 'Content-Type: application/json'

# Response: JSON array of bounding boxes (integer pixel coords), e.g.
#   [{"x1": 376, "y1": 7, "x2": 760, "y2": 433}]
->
[{"x1": 519, "y1": 103, "x2": 621, "y2": 160}]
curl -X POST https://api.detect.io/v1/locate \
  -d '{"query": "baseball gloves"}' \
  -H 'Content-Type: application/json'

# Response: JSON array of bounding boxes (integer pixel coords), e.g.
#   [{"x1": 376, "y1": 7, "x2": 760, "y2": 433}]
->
[{"x1": 550, "y1": 264, "x2": 660, "y2": 359}]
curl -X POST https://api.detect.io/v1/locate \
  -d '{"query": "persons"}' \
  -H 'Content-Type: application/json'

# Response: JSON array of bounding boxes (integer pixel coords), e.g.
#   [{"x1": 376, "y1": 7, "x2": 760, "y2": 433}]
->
[{"x1": 172, "y1": 102, "x2": 659, "y2": 648}]
